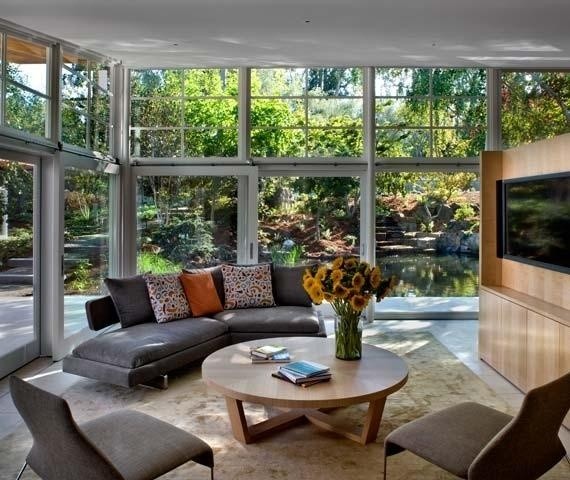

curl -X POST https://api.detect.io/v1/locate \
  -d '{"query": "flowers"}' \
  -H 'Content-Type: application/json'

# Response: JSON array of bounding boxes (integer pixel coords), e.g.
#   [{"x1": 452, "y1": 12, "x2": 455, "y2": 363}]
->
[{"x1": 301, "y1": 253, "x2": 400, "y2": 317}]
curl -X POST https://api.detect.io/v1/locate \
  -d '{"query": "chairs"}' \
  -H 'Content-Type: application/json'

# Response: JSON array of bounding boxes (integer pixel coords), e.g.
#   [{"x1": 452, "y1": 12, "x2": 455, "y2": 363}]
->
[
  {"x1": 5, "y1": 370, "x2": 214, "y2": 480},
  {"x1": 381, "y1": 372, "x2": 570, "y2": 479}
]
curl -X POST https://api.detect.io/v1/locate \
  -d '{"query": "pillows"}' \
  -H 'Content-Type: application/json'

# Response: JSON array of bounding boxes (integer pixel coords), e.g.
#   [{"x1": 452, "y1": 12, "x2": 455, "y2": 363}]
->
[{"x1": 104, "y1": 261, "x2": 319, "y2": 329}]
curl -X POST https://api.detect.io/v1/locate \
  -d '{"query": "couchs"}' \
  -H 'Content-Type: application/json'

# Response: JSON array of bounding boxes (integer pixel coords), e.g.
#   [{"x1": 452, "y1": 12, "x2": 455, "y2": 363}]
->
[{"x1": 61, "y1": 260, "x2": 327, "y2": 391}]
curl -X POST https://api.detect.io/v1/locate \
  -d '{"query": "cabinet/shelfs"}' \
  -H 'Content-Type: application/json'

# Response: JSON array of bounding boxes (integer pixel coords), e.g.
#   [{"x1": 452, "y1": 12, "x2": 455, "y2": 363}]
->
[{"x1": 477, "y1": 133, "x2": 570, "y2": 429}]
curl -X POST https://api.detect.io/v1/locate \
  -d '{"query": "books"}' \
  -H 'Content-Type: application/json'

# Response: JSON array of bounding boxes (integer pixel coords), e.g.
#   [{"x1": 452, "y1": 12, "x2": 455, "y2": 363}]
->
[
  {"x1": 251, "y1": 351, "x2": 290, "y2": 364},
  {"x1": 250, "y1": 343, "x2": 287, "y2": 360},
  {"x1": 280, "y1": 359, "x2": 331, "y2": 379},
  {"x1": 278, "y1": 368, "x2": 332, "y2": 384},
  {"x1": 272, "y1": 372, "x2": 330, "y2": 388}
]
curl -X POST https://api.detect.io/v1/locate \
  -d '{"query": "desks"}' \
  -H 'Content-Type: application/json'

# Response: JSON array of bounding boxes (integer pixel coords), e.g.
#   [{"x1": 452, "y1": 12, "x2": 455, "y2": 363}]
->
[{"x1": 199, "y1": 336, "x2": 409, "y2": 445}]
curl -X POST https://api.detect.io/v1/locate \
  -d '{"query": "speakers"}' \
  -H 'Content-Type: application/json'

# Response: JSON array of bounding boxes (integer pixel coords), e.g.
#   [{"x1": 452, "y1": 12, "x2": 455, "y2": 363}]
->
[{"x1": 496, "y1": 179, "x2": 503, "y2": 259}]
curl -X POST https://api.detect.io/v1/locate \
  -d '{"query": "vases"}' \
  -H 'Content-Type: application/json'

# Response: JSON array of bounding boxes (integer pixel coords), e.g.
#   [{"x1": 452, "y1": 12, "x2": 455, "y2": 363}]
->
[{"x1": 333, "y1": 314, "x2": 363, "y2": 360}]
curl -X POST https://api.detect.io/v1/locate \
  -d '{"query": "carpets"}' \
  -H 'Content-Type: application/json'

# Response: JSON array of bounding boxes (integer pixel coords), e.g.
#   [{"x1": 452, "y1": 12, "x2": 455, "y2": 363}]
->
[{"x1": 0, "y1": 330, "x2": 570, "y2": 480}]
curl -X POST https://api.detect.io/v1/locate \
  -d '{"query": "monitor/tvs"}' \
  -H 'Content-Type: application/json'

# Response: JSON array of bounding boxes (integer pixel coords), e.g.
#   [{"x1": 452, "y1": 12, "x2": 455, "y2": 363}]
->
[{"x1": 502, "y1": 171, "x2": 570, "y2": 275}]
